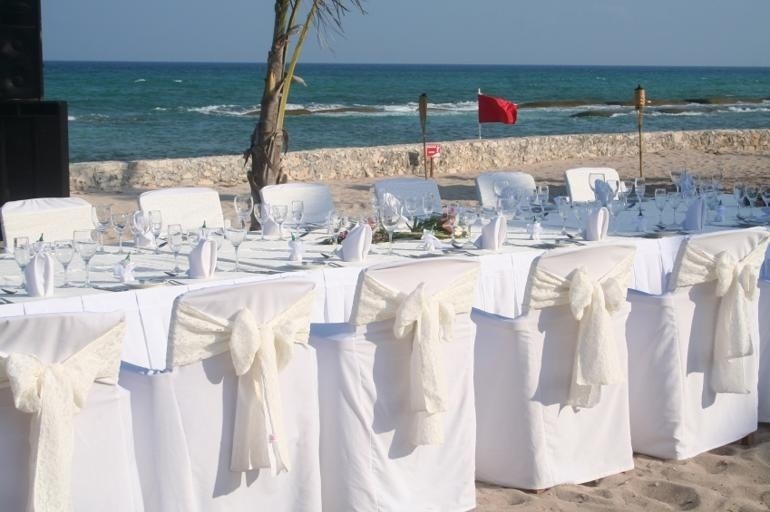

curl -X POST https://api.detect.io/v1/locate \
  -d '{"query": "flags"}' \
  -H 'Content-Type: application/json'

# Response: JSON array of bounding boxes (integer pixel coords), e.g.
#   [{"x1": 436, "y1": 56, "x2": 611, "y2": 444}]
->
[{"x1": 477, "y1": 93, "x2": 518, "y2": 124}]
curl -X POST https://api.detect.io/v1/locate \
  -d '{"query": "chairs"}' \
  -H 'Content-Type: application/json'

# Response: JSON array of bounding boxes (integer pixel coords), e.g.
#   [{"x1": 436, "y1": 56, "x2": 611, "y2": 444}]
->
[{"x1": 0, "y1": 166, "x2": 769, "y2": 512}]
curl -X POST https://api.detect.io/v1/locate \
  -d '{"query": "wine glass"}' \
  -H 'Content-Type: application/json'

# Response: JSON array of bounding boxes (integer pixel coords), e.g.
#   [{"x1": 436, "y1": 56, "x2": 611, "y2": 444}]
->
[{"x1": 13, "y1": 161, "x2": 770, "y2": 289}]
